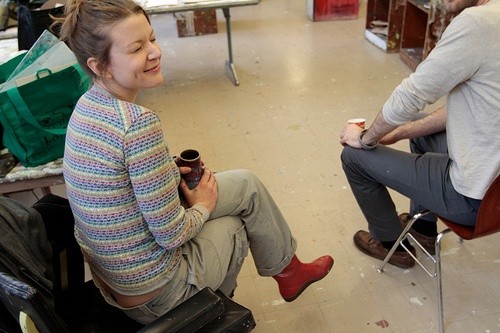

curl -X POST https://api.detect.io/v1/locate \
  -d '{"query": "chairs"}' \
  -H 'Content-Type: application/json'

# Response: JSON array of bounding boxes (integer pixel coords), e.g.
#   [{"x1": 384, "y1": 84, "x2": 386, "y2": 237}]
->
[
  {"x1": 4, "y1": 194, "x2": 257, "y2": 333},
  {"x1": 377, "y1": 173, "x2": 500, "y2": 333}
]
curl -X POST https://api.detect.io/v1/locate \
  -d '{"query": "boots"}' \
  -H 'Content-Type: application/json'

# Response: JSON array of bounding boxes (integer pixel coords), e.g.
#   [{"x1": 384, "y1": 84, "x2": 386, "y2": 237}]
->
[{"x1": 272, "y1": 254, "x2": 334, "y2": 302}]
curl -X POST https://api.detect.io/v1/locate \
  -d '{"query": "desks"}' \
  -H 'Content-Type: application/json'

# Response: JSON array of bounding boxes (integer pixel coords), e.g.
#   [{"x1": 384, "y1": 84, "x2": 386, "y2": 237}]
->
[
  {"x1": 142, "y1": 0, "x2": 259, "y2": 86},
  {"x1": 0, "y1": 146, "x2": 64, "y2": 200}
]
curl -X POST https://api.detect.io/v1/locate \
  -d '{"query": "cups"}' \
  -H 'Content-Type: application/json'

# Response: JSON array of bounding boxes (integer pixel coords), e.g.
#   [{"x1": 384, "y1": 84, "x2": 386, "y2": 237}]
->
[
  {"x1": 348, "y1": 118, "x2": 366, "y2": 129},
  {"x1": 180, "y1": 149, "x2": 204, "y2": 190}
]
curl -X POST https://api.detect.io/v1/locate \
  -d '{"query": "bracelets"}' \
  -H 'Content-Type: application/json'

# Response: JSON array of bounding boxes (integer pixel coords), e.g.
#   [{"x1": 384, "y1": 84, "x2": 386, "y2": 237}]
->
[{"x1": 358, "y1": 128, "x2": 380, "y2": 150}]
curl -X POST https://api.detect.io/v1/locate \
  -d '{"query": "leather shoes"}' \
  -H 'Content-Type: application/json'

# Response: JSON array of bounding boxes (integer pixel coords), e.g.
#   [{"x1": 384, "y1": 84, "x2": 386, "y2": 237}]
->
[
  {"x1": 353, "y1": 230, "x2": 416, "y2": 268},
  {"x1": 398, "y1": 213, "x2": 440, "y2": 255}
]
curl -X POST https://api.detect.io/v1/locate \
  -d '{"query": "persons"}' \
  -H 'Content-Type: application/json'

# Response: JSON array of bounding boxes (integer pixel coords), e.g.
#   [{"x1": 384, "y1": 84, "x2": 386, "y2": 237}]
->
[
  {"x1": 63, "y1": 0, "x2": 334, "y2": 325},
  {"x1": 340, "y1": 0, "x2": 500, "y2": 269}
]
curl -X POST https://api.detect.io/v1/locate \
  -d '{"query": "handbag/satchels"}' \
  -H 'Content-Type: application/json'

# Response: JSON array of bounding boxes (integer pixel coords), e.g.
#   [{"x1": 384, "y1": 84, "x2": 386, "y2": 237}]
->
[{"x1": 0, "y1": 47, "x2": 93, "y2": 168}]
